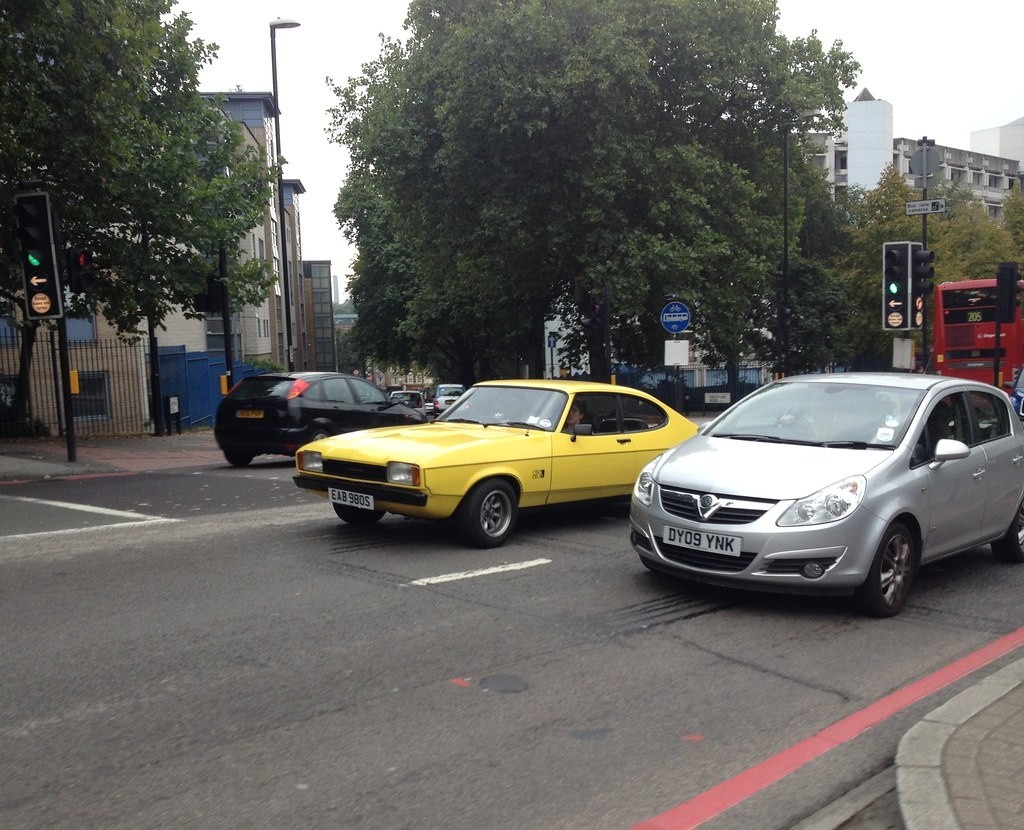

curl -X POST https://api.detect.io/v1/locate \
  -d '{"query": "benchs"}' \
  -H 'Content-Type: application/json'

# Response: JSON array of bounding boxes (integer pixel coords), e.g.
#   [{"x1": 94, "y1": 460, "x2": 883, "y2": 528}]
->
[{"x1": 597, "y1": 418, "x2": 648, "y2": 433}]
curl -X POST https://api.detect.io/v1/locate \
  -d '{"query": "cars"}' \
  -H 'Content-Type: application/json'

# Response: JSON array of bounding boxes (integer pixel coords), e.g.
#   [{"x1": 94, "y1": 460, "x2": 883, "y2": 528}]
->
[
  {"x1": 433, "y1": 383, "x2": 472, "y2": 418},
  {"x1": 293, "y1": 379, "x2": 699, "y2": 549},
  {"x1": 629, "y1": 371, "x2": 1024, "y2": 621},
  {"x1": 389, "y1": 390, "x2": 427, "y2": 417},
  {"x1": 1003, "y1": 364, "x2": 1024, "y2": 422},
  {"x1": 213, "y1": 371, "x2": 429, "y2": 467}
]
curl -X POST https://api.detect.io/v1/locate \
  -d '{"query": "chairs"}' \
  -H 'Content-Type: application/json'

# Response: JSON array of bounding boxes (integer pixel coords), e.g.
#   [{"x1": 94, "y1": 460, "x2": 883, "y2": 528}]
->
[
  {"x1": 918, "y1": 401, "x2": 954, "y2": 461},
  {"x1": 581, "y1": 412, "x2": 598, "y2": 434}
]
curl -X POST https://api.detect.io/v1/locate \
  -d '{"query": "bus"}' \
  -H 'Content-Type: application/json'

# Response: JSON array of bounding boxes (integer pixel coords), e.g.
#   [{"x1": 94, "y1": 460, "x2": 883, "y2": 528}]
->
[{"x1": 934, "y1": 280, "x2": 1024, "y2": 396}]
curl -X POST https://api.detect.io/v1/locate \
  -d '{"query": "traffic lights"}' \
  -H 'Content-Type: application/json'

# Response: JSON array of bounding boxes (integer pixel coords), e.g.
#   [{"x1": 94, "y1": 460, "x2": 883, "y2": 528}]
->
[
  {"x1": 16, "y1": 192, "x2": 64, "y2": 319},
  {"x1": 910, "y1": 241, "x2": 934, "y2": 329},
  {"x1": 882, "y1": 241, "x2": 910, "y2": 330}
]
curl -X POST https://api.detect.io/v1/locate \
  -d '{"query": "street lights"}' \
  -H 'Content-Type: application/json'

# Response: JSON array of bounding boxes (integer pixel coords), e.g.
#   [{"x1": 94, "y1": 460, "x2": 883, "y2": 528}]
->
[{"x1": 270, "y1": 19, "x2": 302, "y2": 371}]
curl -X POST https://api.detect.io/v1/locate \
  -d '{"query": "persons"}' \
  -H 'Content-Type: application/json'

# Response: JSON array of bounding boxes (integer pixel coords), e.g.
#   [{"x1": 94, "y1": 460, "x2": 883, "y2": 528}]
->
[{"x1": 562, "y1": 400, "x2": 594, "y2": 435}]
